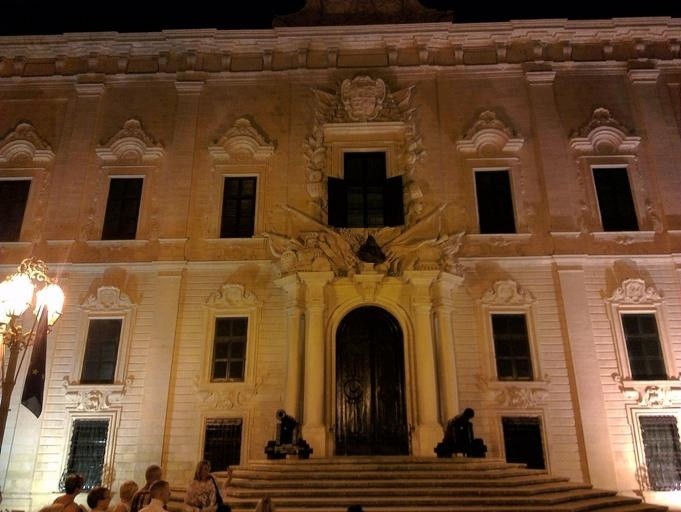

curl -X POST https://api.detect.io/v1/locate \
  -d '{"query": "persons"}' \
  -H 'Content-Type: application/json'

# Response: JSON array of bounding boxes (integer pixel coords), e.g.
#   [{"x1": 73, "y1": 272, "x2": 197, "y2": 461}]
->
[
  {"x1": 130, "y1": 464, "x2": 167, "y2": 512},
  {"x1": 184, "y1": 460, "x2": 223, "y2": 512},
  {"x1": 87, "y1": 486, "x2": 112, "y2": 512},
  {"x1": 53, "y1": 474, "x2": 88, "y2": 512},
  {"x1": 138, "y1": 479, "x2": 171, "y2": 512},
  {"x1": 111, "y1": 480, "x2": 139, "y2": 512}
]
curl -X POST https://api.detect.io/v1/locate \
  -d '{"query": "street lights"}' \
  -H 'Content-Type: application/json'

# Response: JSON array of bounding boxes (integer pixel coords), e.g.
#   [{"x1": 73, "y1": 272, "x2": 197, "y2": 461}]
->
[{"x1": 0, "y1": 257, "x2": 66, "y2": 458}]
